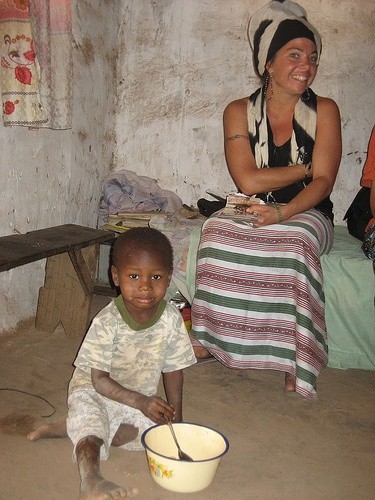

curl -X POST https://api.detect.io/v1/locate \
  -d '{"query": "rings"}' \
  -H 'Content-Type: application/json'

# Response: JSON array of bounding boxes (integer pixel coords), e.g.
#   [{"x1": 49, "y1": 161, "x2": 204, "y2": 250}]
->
[{"x1": 249, "y1": 223, "x2": 254, "y2": 226}]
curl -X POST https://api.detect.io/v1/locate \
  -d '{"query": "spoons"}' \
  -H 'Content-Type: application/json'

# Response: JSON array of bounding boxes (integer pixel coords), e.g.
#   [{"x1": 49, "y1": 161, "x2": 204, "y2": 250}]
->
[{"x1": 163, "y1": 414, "x2": 194, "y2": 461}]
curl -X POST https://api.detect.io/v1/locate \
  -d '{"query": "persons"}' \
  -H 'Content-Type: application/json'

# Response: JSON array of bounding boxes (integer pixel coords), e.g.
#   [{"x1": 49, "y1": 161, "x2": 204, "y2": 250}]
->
[
  {"x1": 359, "y1": 128, "x2": 375, "y2": 276},
  {"x1": 190, "y1": 19, "x2": 344, "y2": 398},
  {"x1": 27, "y1": 226, "x2": 198, "y2": 500}
]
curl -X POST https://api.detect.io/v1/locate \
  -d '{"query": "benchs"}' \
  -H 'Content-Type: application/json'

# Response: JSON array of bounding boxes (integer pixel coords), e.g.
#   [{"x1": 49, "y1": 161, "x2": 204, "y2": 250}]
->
[
  {"x1": 0, "y1": 223, "x2": 116, "y2": 335},
  {"x1": 150, "y1": 213, "x2": 375, "y2": 371}
]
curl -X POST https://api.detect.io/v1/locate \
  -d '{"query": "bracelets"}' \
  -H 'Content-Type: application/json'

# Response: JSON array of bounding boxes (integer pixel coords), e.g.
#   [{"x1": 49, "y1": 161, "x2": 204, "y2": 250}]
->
[
  {"x1": 275, "y1": 206, "x2": 281, "y2": 223},
  {"x1": 304, "y1": 164, "x2": 310, "y2": 179}
]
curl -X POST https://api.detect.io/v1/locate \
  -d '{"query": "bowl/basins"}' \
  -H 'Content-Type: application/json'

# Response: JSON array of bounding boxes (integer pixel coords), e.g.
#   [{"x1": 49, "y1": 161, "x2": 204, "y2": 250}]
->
[{"x1": 141, "y1": 421, "x2": 230, "y2": 494}]
